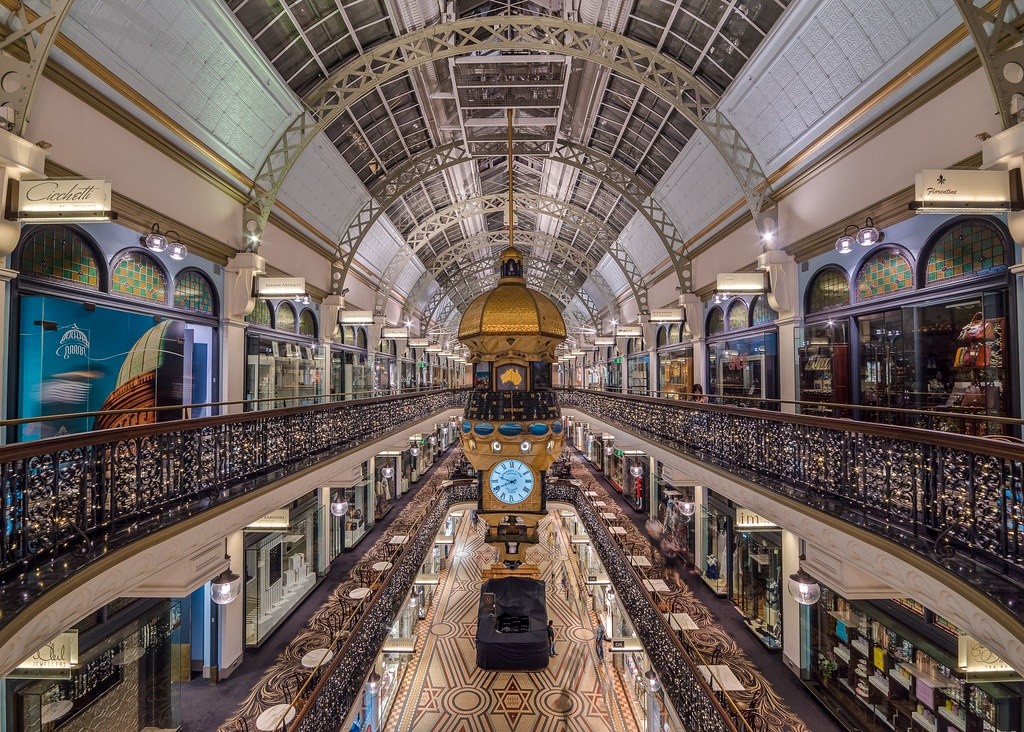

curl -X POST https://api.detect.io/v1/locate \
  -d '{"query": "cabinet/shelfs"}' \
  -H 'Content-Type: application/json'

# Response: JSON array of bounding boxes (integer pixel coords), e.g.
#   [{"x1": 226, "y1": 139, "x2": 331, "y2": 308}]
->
[{"x1": 815, "y1": 583, "x2": 1024, "y2": 732}]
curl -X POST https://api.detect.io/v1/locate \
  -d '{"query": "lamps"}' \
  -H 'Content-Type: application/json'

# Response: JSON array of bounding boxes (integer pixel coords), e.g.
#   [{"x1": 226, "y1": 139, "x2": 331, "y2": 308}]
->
[
  {"x1": 209, "y1": 418, "x2": 456, "y2": 694},
  {"x1": 140, "y1": 223, "x2": 313, "y2": 306},
  {"x1": 568, "y1": 416, "x2": 820, "y2": 692},
  {"x1": 711, "y1": 215, "x2": 885, "y2": 306}
]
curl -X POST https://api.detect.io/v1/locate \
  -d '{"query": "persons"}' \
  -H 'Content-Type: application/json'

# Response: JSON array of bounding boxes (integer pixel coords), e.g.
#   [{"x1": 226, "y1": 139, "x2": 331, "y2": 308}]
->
[
  {"x1": 659, "y1": 489, "x2": 692, "y2": 542},
  {"x1": 636, "y1": 470, "x2": 642, "y2": 509},
  {"x1": 764, "y1": 577, "x2": 778, "y2": 632},
  {"x1": 376, "y1": 477, "x2": 391, "y2": 512},
  {"x1": 720, "y1": 521, "x2": 742, "y2": 587},
  {"x1": 961, "y1": 380, "x2": 982, "y2": 406},
  {"x1": 690, "y1": 384, "x2": 707, "y2": 403}
]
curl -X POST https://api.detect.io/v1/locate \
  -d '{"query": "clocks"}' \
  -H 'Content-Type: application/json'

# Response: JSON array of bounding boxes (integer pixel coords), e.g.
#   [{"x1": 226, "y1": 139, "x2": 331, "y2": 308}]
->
[{"x1": 490, "y1": 459, "x2": 534, "y2": 505}]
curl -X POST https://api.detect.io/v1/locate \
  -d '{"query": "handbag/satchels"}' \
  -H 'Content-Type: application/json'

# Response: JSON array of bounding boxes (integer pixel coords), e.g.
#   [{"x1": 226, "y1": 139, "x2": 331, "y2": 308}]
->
[
  {"x1": 957, "y1": 312, "x2": 994, "y2": 342},
  {"x1": 954, "y1": 344, "x2": 991, "y2": 367},
  {"x1": 952, "y1": 377, "x2": 986, "y2": 407},
  {"x1": 986, "y1": 381, "x2": 1000, "y2": 409}
]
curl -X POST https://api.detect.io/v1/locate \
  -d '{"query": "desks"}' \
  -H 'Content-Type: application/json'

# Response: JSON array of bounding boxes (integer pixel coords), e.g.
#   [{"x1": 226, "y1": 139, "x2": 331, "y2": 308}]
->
[
  {"x1": 256, "y1": 704, "x2": 296, "y2": 731},
  {"x1": 642, "y1": 578, "x2": 670, "y2": 599},
  {"x1": 663, "y1": 613, "x2": 701, "y2": 642},
  {"x1": 302, "y1": 649, "x2": 334, "y2": 674},
  {"x1": 584, "y1": 491, "x2": 599, "y2": 498},
  {"x1": 626, "y1": 556, "x2": 651, "y2": 571},
  {"x1": 389, "y1": 535, "x2": 409, "y2": 548},
  {"x1": 697, "y1": 664, "x2": 746, "y2": 699},
  {"x1": 372, "y1": 562, "x2": 393, "y2": 577},
  {"x1": 608, "y1": 526, "x2": 627, "y2": 538},
  {"x1": 590, "y1": 500, "x2": 607, "y2": 509},
  {"x1": 599, "y1": 512, "x2": 617, "y2": 521},
  {"x1": 348, "y1": 588, "x2": 373, "y2": 607}
]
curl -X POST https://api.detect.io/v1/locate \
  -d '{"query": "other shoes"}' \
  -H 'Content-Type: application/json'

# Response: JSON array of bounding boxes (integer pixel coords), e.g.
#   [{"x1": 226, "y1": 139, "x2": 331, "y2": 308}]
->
[
  {"x1": 599, "y1": 659, "x2": 604, "y2": 664},
  {"x1": 553, "y1": 653, "x2": 558, "y2": 655},
  {"x1": 550, "y1": 655, "x2": 555, "y2": 658}
]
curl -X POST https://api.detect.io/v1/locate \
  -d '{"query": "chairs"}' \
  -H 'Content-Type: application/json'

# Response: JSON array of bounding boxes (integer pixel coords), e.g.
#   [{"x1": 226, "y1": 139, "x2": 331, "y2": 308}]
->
[
  {"x1": 238, "y1": 539, "x2": 406, "y2": 732},
  {"x1": 581, "y1": 478, "x2": 769, "y2": 732}
]
